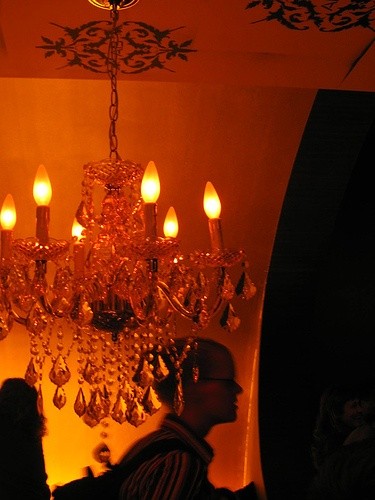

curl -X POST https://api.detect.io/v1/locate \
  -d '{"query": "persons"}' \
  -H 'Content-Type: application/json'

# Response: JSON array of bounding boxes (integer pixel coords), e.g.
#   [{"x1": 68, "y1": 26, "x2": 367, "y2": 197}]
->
[
  {"x1": 115, "y1": 335, "x2": 244, "y2": 500},
  {"x1": 0, "y1": 377, "x2": 52, "y2": 500}
]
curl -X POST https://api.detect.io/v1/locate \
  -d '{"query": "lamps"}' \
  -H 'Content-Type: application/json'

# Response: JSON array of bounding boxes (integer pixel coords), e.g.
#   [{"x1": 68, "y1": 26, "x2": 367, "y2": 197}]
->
[{"x1": 0, "y1": 0, "x2": 257, "y2": 462}]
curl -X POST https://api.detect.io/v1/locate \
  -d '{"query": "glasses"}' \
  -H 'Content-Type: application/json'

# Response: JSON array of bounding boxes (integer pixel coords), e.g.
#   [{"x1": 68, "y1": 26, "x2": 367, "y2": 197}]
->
[{"x1": 194, "y1": 377, "x2": 235, "y2": 386}]
